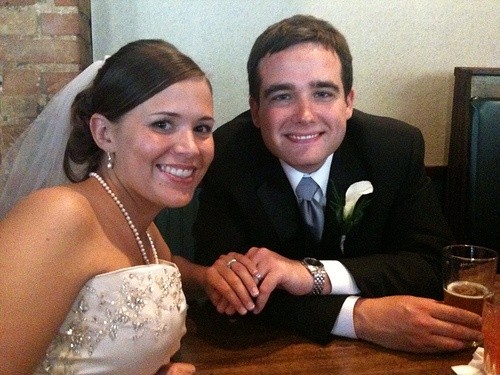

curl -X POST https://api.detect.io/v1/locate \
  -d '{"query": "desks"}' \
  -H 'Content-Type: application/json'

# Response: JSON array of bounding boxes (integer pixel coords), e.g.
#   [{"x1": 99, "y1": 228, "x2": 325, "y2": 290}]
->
[{"x1": 159, "y1": 267, "x2": 500, "y2": 375}]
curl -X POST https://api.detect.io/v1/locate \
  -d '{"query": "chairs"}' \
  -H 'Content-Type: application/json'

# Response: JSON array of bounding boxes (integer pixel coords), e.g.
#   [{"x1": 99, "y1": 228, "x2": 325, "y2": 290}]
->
[{"x1": 449, "y1": 65, "x2": 500, "y2": 246}]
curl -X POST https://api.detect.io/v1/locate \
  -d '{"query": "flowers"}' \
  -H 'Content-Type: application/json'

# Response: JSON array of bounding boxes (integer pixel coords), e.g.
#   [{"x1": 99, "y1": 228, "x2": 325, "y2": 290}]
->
[{"x1": 328, "y1": 175, "x2": 375, "y2": 259}]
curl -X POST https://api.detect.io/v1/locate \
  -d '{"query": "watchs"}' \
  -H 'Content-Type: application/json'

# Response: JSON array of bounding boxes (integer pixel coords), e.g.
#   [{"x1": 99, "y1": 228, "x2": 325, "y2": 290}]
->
[{"x1": 301, "y1": 257, "x2": 326, "y2": 295}]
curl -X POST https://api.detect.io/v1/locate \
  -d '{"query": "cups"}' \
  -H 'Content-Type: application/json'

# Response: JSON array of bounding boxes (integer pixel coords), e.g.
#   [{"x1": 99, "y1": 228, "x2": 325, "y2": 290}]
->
[
  {"x1": 441, "y1": 244, "x2": 497, "y2": 317},
  {"x1": 481, "y1": 290, "x2": 500, "y2": 375}
]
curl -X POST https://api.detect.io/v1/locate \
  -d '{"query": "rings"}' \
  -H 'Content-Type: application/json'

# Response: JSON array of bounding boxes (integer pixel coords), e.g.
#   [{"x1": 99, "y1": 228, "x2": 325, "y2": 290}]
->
[
  {"x1": 227, "y1": 260, "x2": 236, "y2": 267},
  {"x1": 256, "y1": 273, "x2": 262, "y2": 281}
]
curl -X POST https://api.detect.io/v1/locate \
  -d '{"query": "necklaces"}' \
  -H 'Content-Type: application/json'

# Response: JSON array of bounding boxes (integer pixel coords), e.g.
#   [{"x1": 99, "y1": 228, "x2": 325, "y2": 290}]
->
[{"x1": 89, "y1": 172, "x2": 159, "y2": 265}]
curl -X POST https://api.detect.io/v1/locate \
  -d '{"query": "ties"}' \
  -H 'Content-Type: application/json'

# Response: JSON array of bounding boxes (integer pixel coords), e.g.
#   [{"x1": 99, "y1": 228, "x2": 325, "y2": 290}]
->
[{"x1": 296, "y1": 177, "x2": 325, "y2": 240}]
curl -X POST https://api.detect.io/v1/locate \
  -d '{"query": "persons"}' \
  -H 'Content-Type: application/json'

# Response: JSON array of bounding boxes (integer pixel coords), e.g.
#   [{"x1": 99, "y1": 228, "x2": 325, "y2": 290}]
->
[
  {"x1": 154, "y1": 14, "x2": 485, "y2": 354},
  {"x1": 0, "y1": 38, "x2": 216, "y2": 375}
]
